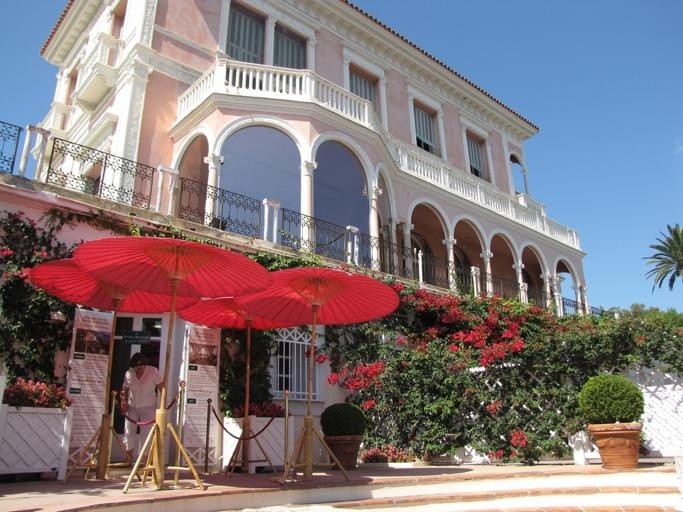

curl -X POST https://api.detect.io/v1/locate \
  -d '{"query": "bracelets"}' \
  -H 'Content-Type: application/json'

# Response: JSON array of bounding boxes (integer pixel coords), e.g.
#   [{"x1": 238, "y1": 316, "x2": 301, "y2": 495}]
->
[{"x1": 121, "y1": 398, "x2": 126, "y2": 401}]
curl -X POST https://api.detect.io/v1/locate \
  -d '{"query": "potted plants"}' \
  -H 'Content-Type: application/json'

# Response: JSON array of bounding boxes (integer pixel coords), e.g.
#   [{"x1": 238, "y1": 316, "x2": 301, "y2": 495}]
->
[
  {"x1": 576, "y1": 371, "x2": 661, "y2": 485},
  {"x1": 318, "y1": 401, "x2": 366, "y2": 470}
]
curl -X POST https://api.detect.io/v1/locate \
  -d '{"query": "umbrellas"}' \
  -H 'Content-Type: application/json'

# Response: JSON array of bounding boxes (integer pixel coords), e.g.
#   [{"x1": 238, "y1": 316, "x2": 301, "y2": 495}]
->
[
  {"x1": 178, "y1": 296, "x2": 292, "y2": 474},
  {"x1": 30, "y1": 256, "x2": 200, "y2": 478},
  {"x1": 73, "y1": 235, "x2": 271, "y2": 489},
  {"x1": 270, "y1": 266, "x2": 401, "y2": 481}
]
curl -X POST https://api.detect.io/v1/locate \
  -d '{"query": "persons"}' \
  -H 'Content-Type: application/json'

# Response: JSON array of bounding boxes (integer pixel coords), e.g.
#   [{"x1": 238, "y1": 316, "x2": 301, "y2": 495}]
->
[{"x1": 118, "y1": 353, "x2": 164, "y2": 476}]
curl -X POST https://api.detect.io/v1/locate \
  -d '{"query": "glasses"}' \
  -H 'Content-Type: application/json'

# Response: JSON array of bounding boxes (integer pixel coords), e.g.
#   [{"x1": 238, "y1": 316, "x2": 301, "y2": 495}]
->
[{"x1": 135, "y1": 363, "x2": 144, "y2": 367}]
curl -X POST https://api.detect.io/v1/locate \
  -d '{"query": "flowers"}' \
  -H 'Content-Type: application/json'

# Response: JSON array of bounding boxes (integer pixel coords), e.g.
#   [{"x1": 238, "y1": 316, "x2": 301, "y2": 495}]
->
[
  {"x1": 226, "y1": 402, "x2": 289, "y2": 423},
  {"x1": 2, "y1": 378, "x2": 69, "y2": 408}
]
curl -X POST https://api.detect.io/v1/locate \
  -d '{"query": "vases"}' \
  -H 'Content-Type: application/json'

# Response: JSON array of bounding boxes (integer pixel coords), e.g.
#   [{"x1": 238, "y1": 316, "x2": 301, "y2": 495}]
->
[
  {"x1": 218, "y1": 415, "x2": 296, "y2": 475},
  {"x1": 0, "y1": 403, "x2": 73, "y2": 482}
]
